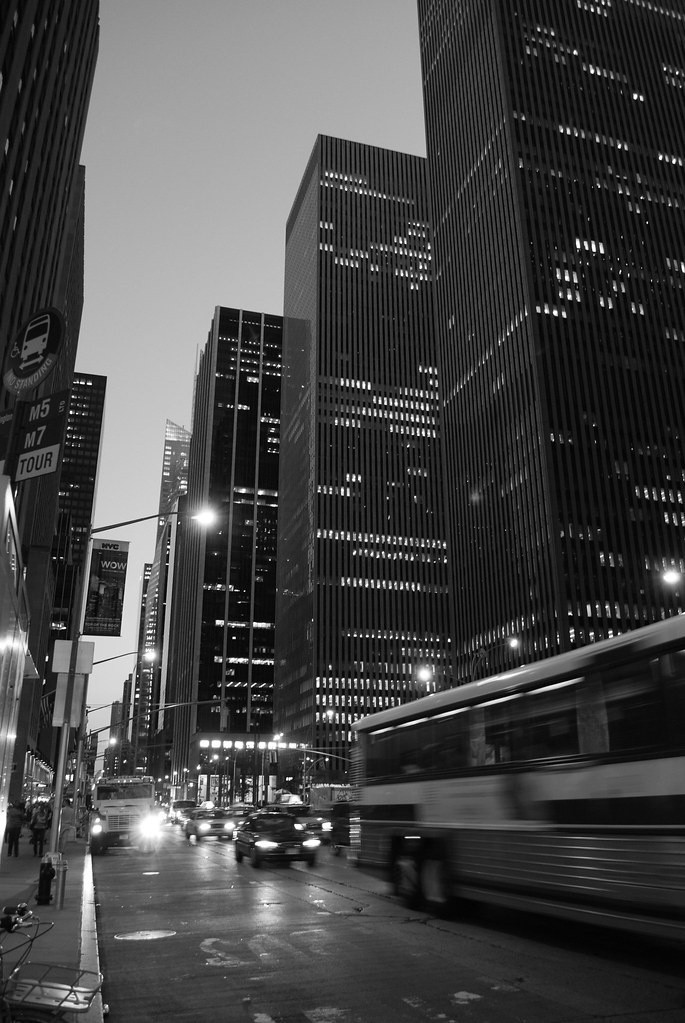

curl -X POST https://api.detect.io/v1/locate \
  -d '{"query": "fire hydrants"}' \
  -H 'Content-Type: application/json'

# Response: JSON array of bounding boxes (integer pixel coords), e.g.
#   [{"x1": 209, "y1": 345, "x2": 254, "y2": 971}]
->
[{"x1": 35, "y1": 855, "x2": 55, "y2": 906}]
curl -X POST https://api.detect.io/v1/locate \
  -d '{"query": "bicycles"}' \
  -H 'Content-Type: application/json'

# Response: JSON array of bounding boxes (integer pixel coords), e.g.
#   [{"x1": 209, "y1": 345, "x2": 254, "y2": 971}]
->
[{"x1": 0, "y1": 902, "x2": 104, "y2": 1023}]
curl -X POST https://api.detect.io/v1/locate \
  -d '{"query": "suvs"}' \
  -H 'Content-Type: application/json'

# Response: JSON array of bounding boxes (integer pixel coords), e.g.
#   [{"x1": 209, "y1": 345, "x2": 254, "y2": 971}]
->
[
  {"x1": 170, "y1": 800, "x2": 199, "y2": 825},
  {"x1": 259, "y1": 805, "x2": 331, "y2": 843},
  {"x1": 330, "y1": 799, "x2": 353, "y2": 854}
]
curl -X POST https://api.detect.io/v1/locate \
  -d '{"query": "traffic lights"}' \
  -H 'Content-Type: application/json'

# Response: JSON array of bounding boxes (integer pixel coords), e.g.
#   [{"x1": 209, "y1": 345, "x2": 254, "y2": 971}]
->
[
  {"x1": 270, "y1": 750, "x2": 278, "y2": 764},
  {"x1": 168, "y1": 748, "x2": 174, "y2": 761},
  {"x1": 220, "y1": 702, "x2": 229, "y2": 729}
]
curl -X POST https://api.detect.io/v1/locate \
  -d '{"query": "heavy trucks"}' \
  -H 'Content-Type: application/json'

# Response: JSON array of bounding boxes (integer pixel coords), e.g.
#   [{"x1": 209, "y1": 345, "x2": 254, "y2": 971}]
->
[{"x1": 89, "y1": 776, "x2": 157, "y2": 856}]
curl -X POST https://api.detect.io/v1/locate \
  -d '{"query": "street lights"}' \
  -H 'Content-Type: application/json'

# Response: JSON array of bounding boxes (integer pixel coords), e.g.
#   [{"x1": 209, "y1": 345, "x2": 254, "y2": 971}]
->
[
  {"x1": 44, "y1": 507, "x2": 219, "y2": 850},
  {"x1": 72, "y1": 650, "x2": 154, "y2": 823}
]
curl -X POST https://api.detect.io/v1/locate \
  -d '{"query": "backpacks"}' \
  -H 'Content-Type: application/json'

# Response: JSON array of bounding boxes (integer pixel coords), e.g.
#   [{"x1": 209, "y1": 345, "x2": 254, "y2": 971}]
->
[{"x1": 34, "y1": 814, "x2": 47, "y2": 829}]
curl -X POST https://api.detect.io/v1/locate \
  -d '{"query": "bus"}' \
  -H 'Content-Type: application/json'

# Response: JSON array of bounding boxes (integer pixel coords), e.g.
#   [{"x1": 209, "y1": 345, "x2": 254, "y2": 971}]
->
[{"x1": 351, "y1": 610, "x2": 685, "y2": 944}]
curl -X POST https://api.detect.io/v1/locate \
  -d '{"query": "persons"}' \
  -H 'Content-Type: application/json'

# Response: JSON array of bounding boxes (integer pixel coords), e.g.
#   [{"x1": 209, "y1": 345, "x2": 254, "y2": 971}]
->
[{"x1": 3, "y1": 798, "x2": 73, "y2": 858}]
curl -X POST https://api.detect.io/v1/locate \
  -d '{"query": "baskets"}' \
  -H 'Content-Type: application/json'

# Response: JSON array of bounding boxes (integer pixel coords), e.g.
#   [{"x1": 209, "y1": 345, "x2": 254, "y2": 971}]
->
[{"x1": 4, "y1": 960, "x2": 104, "y2": 1013}]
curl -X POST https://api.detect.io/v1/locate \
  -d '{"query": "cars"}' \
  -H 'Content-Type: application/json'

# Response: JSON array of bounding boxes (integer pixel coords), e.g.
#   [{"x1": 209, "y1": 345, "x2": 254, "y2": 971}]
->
[
  {"x1": 233, "y1": 813, "x2": 321, "y2": 868},
  {"x1": 186, "y1": 811, "x2": 234, "y2": 842},
  {"x1": 216, "y1": 804, "x2": 259, "y2": 827},
  {"x1": 154, "y1": 792, "x2": 173, "y2": 827}
]
